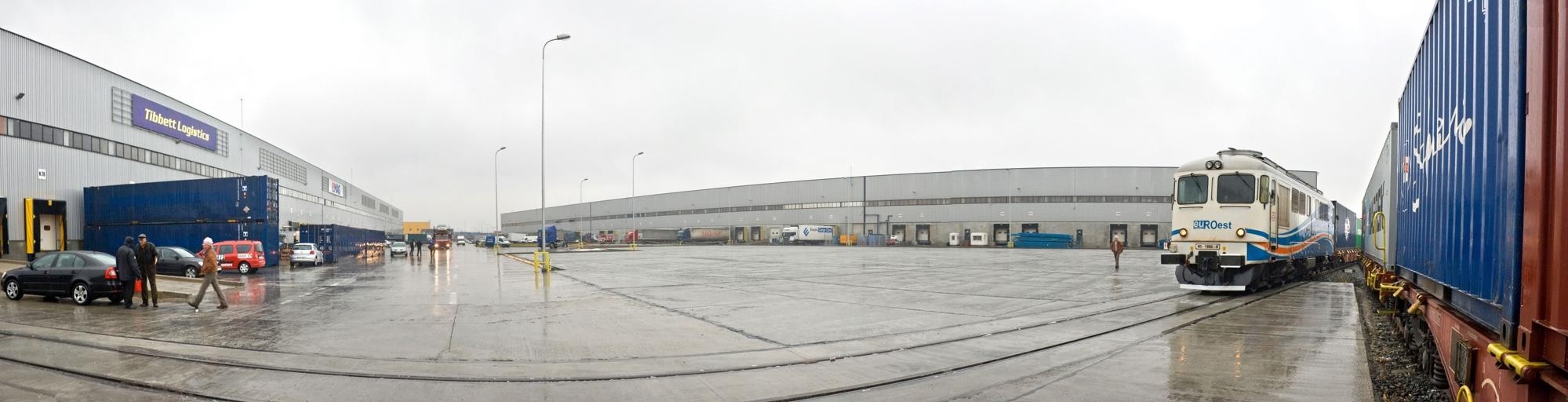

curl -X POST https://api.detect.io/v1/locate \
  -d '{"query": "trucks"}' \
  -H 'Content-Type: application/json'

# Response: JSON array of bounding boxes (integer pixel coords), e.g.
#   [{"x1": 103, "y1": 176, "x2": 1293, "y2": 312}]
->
[
  {"x1": 484, "y1": 235, "x2": 511, "y2": 248},
  {"x1": 407, "y1": 233, "x2": 432, "y2": 244},
  {"x1": 624, "y1": 226, "x2": 679, "y2": 243},
  {"x1": 536, "y1": 224, "x2": 569, "y2": 250},
  {"x1": 431, "y1": 226, "x2": 452, "y2": 250},
  {"x1": 776, "y1": 223, "x2": 836, "y2": 244},
  {"x1": 520, "y1": 236, "x2": 538, "y2": 243},
  {"x1": 676, "y1": 225, "x2": 730, "y2": 242}
]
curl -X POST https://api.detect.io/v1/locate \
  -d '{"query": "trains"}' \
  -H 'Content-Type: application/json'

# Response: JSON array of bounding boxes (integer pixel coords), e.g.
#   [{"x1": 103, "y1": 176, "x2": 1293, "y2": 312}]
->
[
  {"x1": 1154, "y1": 144, "x2": 1364, "y2": 296},
  {"x1": 1362, "y1": 0, "x2": 1565, "y2": 402}
]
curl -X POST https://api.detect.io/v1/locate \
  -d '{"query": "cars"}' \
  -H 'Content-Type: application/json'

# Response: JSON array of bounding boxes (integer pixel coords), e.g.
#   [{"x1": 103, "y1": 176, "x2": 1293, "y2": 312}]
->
[
  {"x1": 154, "y1": 244, "x2": 224, "y2": 279},
  {"x1": 288, "y1": 242, "x2": 326, "y2": 268},
  {"x1": 383, "y1": 239, "x2": 391, "y2": 248},
  {"x1": 389, "y1": 241, "x2": 409, "y2": 258}
]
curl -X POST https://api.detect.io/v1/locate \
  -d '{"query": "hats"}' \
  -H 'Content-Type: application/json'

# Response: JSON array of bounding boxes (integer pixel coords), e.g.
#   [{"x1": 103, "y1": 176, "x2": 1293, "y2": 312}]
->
[
  {"x1": 138, "y1": 233, "x2": 146, "y2": 238},
  {"x1": 204, "y1": 237, "x2": 212, "y2": 246}
]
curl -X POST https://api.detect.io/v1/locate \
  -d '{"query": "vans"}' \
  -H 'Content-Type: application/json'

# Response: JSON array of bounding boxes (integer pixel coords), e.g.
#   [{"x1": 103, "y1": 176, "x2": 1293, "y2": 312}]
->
[
  {"x1": 194, "y1": 238, "x2": 267, "y2": 276},
  {"x1": 456, "y1": 234, "x2": 466, "y2": 246}
]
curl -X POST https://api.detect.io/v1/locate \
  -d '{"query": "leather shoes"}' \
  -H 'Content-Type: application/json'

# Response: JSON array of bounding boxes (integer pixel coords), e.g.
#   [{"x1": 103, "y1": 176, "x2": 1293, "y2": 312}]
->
[
  {"x1": 217, "y1": 305, "x2": 227, "y2": 309},
  {"x1": 188, "y1": 302, "x2": 198, "y2": 309},
  {"x1": 129, "y1": 303, "x2": 158, "y2": 309}
]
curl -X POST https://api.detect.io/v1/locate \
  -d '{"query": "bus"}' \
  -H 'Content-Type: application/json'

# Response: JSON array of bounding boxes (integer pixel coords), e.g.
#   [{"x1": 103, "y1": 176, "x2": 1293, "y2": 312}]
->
[{"x1": 0, "y1": 248, "x2": 140, "y2": 306}]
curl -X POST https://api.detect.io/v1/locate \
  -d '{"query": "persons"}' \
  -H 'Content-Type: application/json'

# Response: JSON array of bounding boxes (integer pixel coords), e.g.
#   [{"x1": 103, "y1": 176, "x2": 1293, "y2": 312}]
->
[
  {"x1": 428, "y1": 237, "x2": 435, "y2": 257},
  {"x1": 134, "y1": 233, "x2": 159, "y2": 307},
  {"x1": 188, "y1": 236, "x2": 229, "y2": 309},
  {"x1": 116, "y1": 236, "x2": 142, "y2": 309},
  {"x1": 409, "y1": 239, "x2": 415, "y2": 256},
  {"x1": 417, "y1": 240, "x2": 422, "y2": 256},
  {"x1": 1111, "y1": 235, "x2": 1124, "y2": 269}
]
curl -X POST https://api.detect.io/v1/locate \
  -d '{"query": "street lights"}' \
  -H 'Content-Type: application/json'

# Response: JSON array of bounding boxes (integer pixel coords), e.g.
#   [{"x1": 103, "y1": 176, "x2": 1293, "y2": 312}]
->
[
  {"x1": 495, "y1": 146, "x2": 507, "y2": 246},
  {"x1": 580, "y1": 177, "x2": 590, "y2": 244},
  {"x1": 538, "y1": 32, "x2": 571, "y2": 272},
  {"x1": 630, "y1": 150, "x2": 645, "y2": 251}
]
